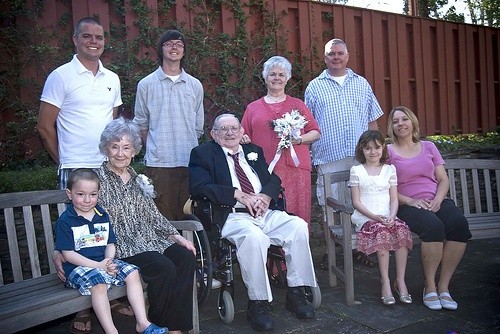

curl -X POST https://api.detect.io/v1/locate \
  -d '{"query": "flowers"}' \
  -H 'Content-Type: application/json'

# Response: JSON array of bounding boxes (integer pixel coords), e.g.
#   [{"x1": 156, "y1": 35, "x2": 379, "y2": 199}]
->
[{"x1": 246, "y1": 108, "x2": 311, "y2": 166}]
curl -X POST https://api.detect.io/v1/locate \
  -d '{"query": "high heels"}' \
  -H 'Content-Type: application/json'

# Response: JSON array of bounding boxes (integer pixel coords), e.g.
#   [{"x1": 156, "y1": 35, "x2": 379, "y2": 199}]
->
[
  {"x1": 381, "y1": 283, "x2": 395, "y2": 305},
  {"x1": 392, "y1": 280, "x2": 412, "y2": 304}
]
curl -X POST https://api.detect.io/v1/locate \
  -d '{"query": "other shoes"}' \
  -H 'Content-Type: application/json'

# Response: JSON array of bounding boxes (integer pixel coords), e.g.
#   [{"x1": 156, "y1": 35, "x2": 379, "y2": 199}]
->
[
  {"x1": 436, "y1": 288, "x2": 457, "y2": 310},
  {"x1": 423, "y1": 287, "x2": 442, "y2": 310}
]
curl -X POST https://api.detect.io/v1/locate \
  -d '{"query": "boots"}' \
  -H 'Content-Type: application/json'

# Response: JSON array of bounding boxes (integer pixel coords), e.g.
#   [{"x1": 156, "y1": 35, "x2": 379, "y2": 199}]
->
[
  {"x1": 247, "y1": 300, "x2": 275, "y2": 332},
  {"x1": 286, "y1": 287, "x2": 315, "y2": 319}
]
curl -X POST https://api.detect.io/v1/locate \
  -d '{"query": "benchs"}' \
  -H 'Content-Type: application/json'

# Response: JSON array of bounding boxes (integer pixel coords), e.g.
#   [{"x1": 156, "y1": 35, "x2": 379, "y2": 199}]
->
[
  {"x1": 0, "y1": 188, "x2": 204, "y2": 334},
  {"x1": 322, "y1": 157, "x2": 500, "y2": 307}
]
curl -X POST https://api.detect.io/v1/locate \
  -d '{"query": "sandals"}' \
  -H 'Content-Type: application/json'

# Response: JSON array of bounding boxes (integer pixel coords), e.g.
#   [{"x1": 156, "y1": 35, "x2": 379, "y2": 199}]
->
[
  {"x1": 321, "y1": 253, "x2": 328, "y2": 271},
  {"x1": 110, "y1": 300, "x2": 135, "y2": 319},
  {"x1": 353, "y1": 252, "x2": 374, "y2": 268},
  {"x1": 70, "y1": 313, "x2": 92, "y2": 333}
]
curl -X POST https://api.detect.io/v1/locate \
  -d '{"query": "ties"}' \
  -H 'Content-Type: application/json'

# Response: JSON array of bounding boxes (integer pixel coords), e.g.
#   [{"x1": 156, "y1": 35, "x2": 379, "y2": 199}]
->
[{"x1": 227, "y1": 153, "x2": 257, "y2": 219}]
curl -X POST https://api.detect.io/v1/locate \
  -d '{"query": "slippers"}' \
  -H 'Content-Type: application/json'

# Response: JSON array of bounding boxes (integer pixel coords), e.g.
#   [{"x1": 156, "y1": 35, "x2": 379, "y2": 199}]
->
[{"x1": 139, "y1": 323, "x2": 169, "y2": 334}]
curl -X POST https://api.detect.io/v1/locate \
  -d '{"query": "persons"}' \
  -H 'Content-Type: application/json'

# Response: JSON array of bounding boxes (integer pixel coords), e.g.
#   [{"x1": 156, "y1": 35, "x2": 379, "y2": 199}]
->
[
  {"x1": 36, "y1": 16, "x2": 135, "y2": 334},
  {"x1": 134, "y1": 29, "x2": 204, "y2": 220},
  {"x1": 51, "y1": 117, "x2": 197, "y2": 334},
  {"x1": 386, "y1": 106, "x2": 469, "y2": 310},
  {"x1": 304, "y1": 37, "x2": 378, "y2": 270},
  {"x1": 349, "y1": 129, "x2": 412, "y2": 306},
  {"x1": 188, "y1": 114, "x2": 316, "y2": 330},
  {"x1": 238, "y1": 56, "x2": 320, "y2": 287},
  {"x1": 55, "y1": 167, "x2": 169, "y2": 334}
]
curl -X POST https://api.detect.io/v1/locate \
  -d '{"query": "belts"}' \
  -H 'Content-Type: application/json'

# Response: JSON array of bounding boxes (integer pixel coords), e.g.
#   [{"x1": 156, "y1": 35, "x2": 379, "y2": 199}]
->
[{"x1": 230, "y1": 208, "x2": 249, "y2": 213}]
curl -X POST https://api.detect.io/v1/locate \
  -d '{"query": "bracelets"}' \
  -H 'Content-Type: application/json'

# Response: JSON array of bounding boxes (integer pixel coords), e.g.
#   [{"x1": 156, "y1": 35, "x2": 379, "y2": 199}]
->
[{"x1": 297, "y1": 138, "x2": 302, "y2": 145}]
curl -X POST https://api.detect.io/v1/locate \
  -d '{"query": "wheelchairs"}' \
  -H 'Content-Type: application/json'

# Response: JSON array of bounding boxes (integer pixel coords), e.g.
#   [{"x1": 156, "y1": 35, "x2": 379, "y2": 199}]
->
[{"x1": 177, "y1": 185, "x2": 321, "y2": 324}]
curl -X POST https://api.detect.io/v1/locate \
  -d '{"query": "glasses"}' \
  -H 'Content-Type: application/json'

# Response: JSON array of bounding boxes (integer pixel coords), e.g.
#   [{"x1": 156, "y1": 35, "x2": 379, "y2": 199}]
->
[
  {"x1": 163, "y1": 42, "x2": 185, "y2": 49},
  {"x1": 214, "y1": 125, "x2": 240, "y2": 133}
]
